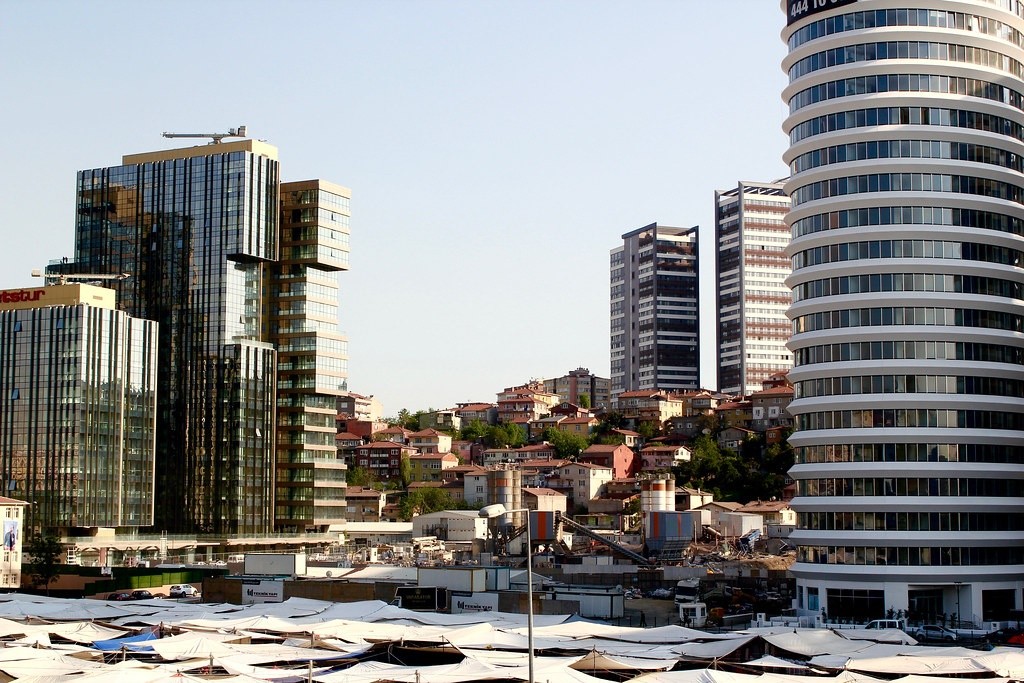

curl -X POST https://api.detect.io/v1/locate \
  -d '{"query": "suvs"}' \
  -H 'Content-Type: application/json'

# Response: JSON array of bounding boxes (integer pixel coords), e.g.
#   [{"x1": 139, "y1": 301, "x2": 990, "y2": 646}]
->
[{"x1": 169, "y1": 584, "x2": 198, "y2": 597}]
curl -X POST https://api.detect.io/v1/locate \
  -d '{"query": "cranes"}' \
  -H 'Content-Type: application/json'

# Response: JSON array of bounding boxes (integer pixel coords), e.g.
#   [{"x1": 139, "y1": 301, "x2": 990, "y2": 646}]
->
[{"x1": 161, "y1": 125, "x2": 246, "y2": 145}]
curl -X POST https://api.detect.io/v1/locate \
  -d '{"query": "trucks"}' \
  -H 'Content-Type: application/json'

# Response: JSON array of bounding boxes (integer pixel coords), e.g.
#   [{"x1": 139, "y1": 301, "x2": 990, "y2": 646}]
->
[{"x1": 228, "y1": 555, "x2": 245, "y2": 564}]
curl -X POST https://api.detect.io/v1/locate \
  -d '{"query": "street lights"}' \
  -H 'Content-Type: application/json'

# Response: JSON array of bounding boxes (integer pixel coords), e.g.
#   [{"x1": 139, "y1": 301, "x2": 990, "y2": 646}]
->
[
  {"x1": 478, "y1": 503, "x2": 534, "y2": 683},
  {"x1": 955, "y1": 581, "x2": 962, "y2": 629}
]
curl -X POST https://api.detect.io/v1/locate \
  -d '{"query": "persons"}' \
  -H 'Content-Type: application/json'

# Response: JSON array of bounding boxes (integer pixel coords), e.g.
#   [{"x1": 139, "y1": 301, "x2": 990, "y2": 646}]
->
[
  {"x1": 682, "y1": 611, "x2": 689, "y2": 626},
  {"x1": 639, "y1": 611, "x2": 647, "y2": 628}
]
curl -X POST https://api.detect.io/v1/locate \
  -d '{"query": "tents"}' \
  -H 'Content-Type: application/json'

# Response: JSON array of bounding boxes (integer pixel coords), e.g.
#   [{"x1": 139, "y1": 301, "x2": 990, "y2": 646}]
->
[{"x1": 0, "y1": 589, "x2": 1024, "y2": 683}]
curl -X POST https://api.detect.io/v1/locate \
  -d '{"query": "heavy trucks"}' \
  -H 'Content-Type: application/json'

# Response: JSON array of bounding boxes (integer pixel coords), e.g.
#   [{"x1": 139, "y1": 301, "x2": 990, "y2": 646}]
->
[{"x1": 675, "y1": 579, "x2": 701, "y2": 608}]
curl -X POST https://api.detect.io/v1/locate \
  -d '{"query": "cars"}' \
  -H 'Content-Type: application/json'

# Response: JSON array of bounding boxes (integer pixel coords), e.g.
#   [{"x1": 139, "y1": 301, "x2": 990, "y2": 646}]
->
[
  {"x1": 193, "y1": 562, "x2": 206, "y2": 565},
  {"x1": 108, "y1": 592, "x2": 135, "y2": 601},
  {"x1": 916, "y1": 625, "x2": 957, "y2": 642},
  {"x1": 308, "y1": 552, "x2": 327, "y2": 562},
  {"x1": 209, "y1": 559, "x2": 227, "y2": 568}
]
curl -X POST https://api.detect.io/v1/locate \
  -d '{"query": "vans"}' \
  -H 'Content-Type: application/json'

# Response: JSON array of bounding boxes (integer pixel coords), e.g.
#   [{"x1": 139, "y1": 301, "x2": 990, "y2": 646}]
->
[
  {"x1": 133, "y1": 590, "x2": 153, "y2": 599},
  {"x1": 154, "y1": 564, "x2": 186, "y2": 568},
  {"x1": 864, "y1": 620, "x2": 902, "y2": 632}
]
